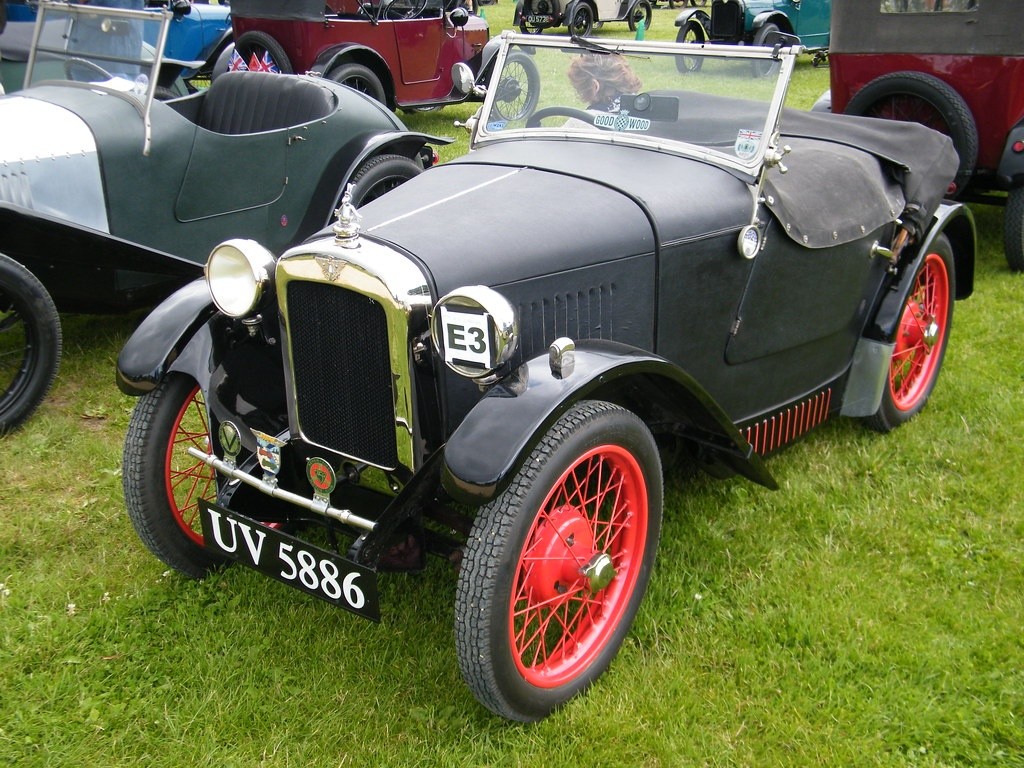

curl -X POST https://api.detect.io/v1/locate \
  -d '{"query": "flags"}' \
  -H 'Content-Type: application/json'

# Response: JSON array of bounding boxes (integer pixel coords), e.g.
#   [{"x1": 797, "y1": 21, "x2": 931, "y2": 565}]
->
[
  {"x1": 249, "y1": 54, "x2": 260, "y2": 71},
  {"x1": 228, "y1": 48, "x2": 246, "y2": 72},
  {"x1": 257, "y1": 51, "x2": 278, "y2": 74}
]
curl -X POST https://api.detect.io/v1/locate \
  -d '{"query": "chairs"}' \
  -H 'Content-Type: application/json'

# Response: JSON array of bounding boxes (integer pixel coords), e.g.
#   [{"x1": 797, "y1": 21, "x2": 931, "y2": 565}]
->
[{"x1": 201, "y1": 70, "x2": 333, "y2": 133}]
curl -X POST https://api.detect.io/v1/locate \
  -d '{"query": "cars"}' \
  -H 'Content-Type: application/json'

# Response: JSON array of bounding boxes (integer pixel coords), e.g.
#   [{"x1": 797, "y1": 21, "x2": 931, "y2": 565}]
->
[
  {"x1": 0, "y1": 2, "x2": 1023, "y2": 443},
  {"x1": 112, "y1": 25, "x2": 979, "y2": 728}
]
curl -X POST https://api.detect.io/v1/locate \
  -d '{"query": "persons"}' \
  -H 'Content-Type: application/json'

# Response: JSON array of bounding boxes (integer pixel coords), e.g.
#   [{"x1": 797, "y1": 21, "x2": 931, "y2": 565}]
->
[
  {"x1": 0, "y1": 0, "x2": 7, "y2": 96},
  {"x1": 67, "y1": 0, "x2": 145, "y2": 83},
  {"x1": 568, "y1": 53, "x2": 641, "y2": 112}
]
